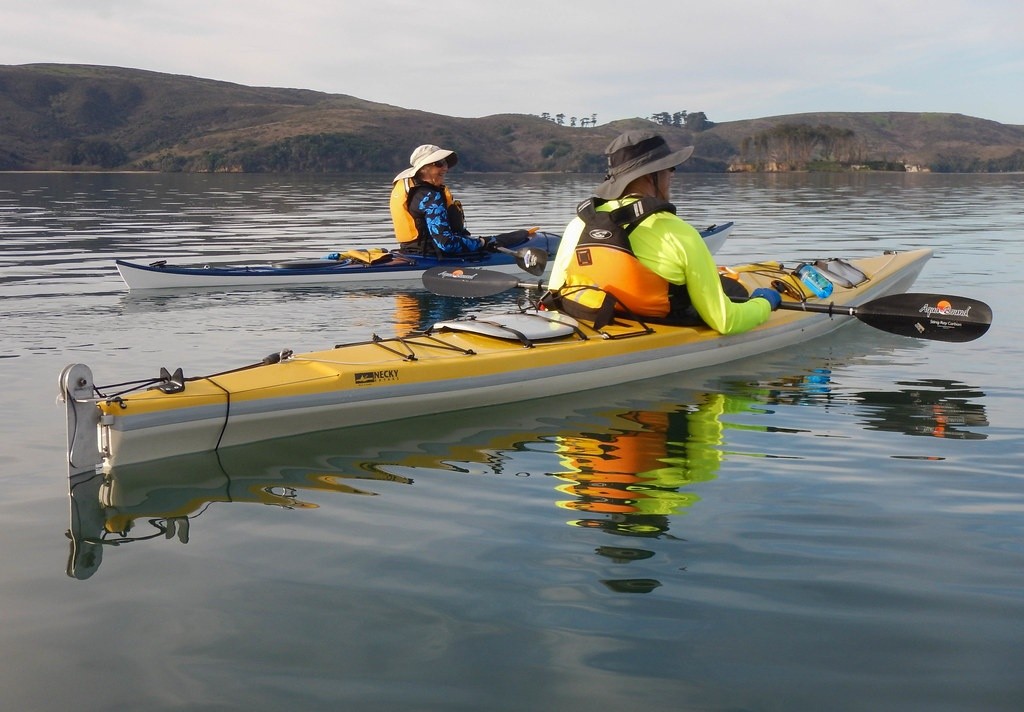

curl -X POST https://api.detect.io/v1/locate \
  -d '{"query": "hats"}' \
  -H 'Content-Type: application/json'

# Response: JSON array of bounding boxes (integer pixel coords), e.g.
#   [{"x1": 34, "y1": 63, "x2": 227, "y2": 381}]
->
[
  {"x1": 392, "y1": 143, "x2": 458, "y2": 187},
  {"x1": 592, "y1": 129, "x2": 695, "y2": 201}
]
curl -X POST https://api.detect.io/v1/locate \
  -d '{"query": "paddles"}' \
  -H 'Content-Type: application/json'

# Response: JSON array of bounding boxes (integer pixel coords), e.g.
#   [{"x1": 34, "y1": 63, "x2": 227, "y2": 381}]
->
[
  {"x1": 465, "y1": 227, "x2": 546, "y2": 276},
  {"x1": 422, "y1": 263, "x2": 994, "y2": 344}
]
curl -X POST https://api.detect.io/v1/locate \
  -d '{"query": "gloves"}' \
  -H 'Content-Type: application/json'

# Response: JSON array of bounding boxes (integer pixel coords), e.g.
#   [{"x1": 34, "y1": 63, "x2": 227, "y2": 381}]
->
[
  {"x1": 749, "y1": 287, "x2": 782, "y2": 311},
  {"x1": 479, "y1": 235, "x2": 504, "y2": 252}
]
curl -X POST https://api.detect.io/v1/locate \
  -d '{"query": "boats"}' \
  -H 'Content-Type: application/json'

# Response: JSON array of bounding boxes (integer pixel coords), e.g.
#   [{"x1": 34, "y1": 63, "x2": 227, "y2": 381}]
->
[{"x1": 52, "y1": 215, "x2": 941, "y2": 483}]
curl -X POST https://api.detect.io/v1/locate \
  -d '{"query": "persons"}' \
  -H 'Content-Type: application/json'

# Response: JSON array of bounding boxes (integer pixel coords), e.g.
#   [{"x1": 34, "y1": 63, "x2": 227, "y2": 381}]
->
[
  {"x1": 539, "y1": 130, "x2": 782, "y2": 336},
  {"x1": 390, "y1": 144, "x2": 506, "y2": 256}
]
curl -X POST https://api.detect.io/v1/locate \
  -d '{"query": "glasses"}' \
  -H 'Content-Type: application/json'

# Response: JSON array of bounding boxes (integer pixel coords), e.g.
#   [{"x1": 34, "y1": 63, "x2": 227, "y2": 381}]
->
[
  {"x1": 665, "y1": 167, "x2": 676, "y2": 172},
  {"x1": 431, "y1": 161, "x2": 449, "y2": 169}
]
